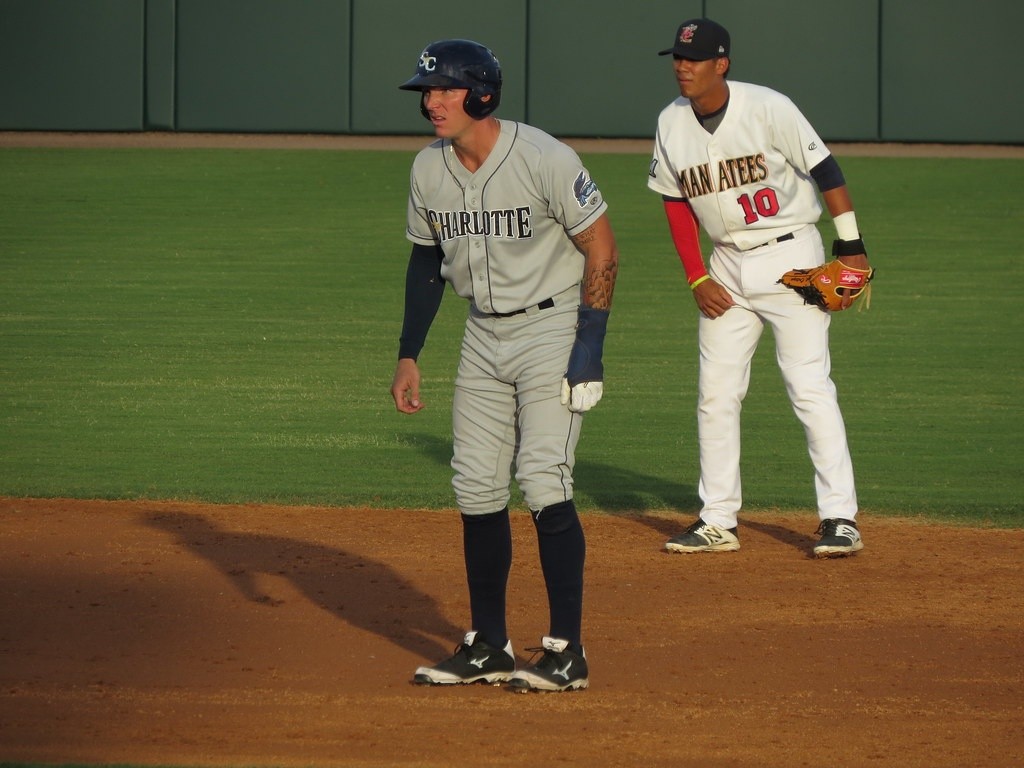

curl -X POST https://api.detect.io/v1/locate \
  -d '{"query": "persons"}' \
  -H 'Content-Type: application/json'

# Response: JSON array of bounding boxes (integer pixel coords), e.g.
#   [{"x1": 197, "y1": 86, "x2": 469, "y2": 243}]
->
[
  {"x1": 389, "y1": 39, "x2": 621, "y2": 694},
  {"x1": 646, "y1": 18, "x2": 874, "y2": 555}
]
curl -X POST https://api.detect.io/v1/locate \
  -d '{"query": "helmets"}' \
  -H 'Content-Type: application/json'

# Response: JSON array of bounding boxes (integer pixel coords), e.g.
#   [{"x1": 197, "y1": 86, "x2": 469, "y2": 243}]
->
[{"x1": 398, "y1": 39, "x2": 503, "y2": 121}]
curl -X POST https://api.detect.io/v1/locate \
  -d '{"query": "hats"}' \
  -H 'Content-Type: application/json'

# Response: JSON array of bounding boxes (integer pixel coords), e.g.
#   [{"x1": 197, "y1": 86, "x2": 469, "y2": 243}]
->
[{"x1": 658, "y1": 18, "x2": 730, "y2": 62}]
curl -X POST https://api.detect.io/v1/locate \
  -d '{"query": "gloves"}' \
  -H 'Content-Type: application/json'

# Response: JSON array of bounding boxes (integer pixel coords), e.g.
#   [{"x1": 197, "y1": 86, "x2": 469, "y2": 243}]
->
[{"x1": 560, "y1": 305, "x2": 610, "y2": 413}]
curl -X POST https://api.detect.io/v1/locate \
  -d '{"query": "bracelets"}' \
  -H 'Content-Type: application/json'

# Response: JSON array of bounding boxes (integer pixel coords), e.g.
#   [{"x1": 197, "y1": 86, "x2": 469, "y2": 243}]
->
[{"x1": 690, "y1": 275, "x2": 712, "y2": 290}]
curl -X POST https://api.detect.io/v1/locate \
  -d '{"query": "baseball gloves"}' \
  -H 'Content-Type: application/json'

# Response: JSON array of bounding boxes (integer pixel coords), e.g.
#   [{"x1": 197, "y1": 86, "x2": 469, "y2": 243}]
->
[{"x1": 782, "y1": 259, "x2": 874, "y2": 312}]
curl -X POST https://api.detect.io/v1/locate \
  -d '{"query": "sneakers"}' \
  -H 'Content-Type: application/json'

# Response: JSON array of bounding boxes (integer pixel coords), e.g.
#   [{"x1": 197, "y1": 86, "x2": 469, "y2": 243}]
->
[
  {"x1": 512, "y1": 636, "x2": 589, "y2": 693},
  {"x1": 666, "y1": 518, "x2": 740, "y2": 555},
  {"x1": 814, "y1": 518, "x2": 863, "y2": 559},
  {"x1": 415, "y1": 632, "x2": 516, "y2": 685}
]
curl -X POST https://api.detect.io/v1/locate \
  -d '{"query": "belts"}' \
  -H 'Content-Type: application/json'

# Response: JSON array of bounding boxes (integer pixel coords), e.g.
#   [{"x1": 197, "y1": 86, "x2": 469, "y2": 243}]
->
[
  {"x1": 488, "y1": 298, "x2": 554, "y2": 317},
  {"x1": 748, "y1": 232, "x2": 794, "y2": 250}
]
